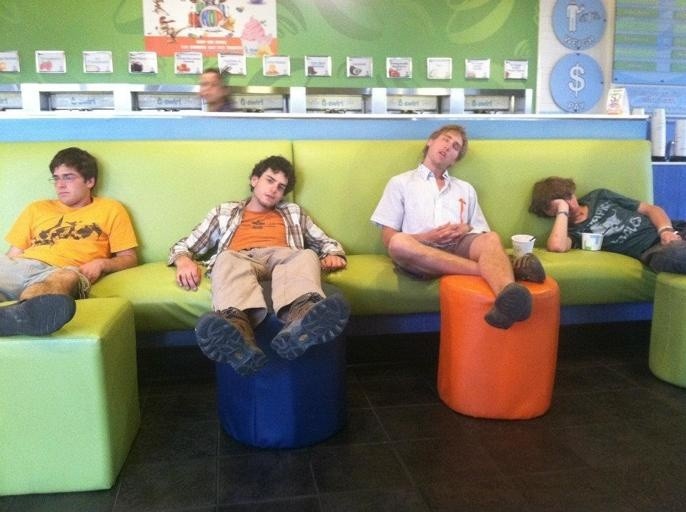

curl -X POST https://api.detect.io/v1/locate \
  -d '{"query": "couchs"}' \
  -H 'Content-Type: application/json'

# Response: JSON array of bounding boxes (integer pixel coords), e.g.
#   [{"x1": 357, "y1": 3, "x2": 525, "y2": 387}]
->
[{"x1": 0, "y1": 115, "x2": 654, "y2": 352}]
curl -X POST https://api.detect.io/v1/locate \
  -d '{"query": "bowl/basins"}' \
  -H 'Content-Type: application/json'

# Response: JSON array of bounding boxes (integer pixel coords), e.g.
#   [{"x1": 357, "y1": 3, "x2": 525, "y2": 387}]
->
[{"x1": 582, "y1": 233, "x2": 605, "y2": 251}]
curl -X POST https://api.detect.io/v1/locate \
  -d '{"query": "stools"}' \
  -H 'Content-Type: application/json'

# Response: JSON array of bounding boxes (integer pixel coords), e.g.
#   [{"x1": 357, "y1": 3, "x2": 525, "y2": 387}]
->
[
  {"x1": 212, "y1": 278, "x2": 346, "y2": 452},
  {"x1": 647, "y1": 270, "x2": 686, "y2": 389},
  {"x1": 0, "y1": 295, "x2": 144, "y2": 498},
  {"x1": 435, "y1": 272, "x2": 561, "y2": 422}
]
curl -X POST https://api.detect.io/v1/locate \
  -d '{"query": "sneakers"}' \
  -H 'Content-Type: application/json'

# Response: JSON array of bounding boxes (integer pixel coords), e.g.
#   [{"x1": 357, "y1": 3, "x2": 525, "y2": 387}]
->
[
  {"x1": 484, "y1": 281, "x2": 532, "y2": 331},
  {"x1": 0, "y1": 293, "x2": 77, "y2": 338},
  {"x1": 507, "y1": 253, "x2": 546, "y2": 283}
]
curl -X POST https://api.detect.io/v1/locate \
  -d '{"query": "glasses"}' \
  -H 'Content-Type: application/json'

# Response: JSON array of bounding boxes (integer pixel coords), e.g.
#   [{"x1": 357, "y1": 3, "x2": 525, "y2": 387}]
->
[{"x1": 47, "y1": 174, "x2": 85, "y2": 183}]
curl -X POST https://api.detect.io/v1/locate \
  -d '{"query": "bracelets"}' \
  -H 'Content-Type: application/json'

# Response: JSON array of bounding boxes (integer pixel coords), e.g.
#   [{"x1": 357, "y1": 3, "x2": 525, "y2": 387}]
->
[
  {"x1": 657, "y1": 226, "x2": 674, "y2": 237},
  {"x1": 555, "y1": 211, "x2": 570, "y2": 216}
]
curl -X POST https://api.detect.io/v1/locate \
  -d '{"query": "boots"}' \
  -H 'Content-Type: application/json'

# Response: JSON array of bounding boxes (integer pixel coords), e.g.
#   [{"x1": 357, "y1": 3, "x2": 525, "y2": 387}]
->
[
  {"x1": 193, "y1": 306, "x2": 269, "y2": 379},
  {"x1": 270, "y1": 291, "x2": 351, "y2": 362}
]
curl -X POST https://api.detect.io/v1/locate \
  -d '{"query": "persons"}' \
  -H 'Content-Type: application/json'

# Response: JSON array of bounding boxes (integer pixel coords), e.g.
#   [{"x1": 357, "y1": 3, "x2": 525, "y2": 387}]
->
[
  {"x1": 198, "y1": 67, "x2": 237, "y2": 111},
  {"x1": 529, "y1": 176, "x2": 686, "y2": 276},
  {"x1": 167, "y1": 155, "x2": 351, "y2": 376},
  {"x1": 0, "y1": 147, "x2": 141, "y2": 337},
  {"x1": 369, "y1": 125, "x2": 546, "y2": 330}
]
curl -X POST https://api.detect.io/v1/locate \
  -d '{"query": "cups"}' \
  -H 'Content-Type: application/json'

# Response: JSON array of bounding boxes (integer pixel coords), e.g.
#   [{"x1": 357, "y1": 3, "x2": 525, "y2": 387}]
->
[
  {"x1": 650, "y1": 108, "x2": 686, "y2": 156},
  {"x1": 511, "y1": 234, "x2": 537, "y2": 259}
]
